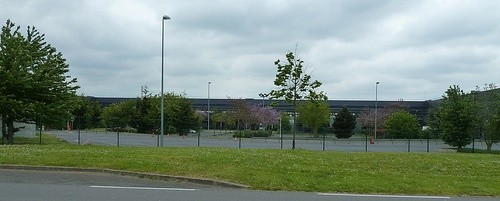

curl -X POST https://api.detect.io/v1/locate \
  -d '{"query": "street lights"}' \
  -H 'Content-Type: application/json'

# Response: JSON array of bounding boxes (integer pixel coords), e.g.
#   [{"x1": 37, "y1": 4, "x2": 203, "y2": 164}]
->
[
  {"x1": 375, "y1": 82, "x2": 380, "y2": 139},
  {"x1": 160, "y1": 15, "x2": 171, "y2": 147},
  {"x1": 207, "y1": 82, "x2": 211, "y2": 130}
]
